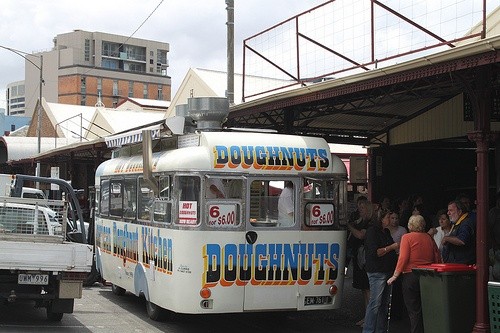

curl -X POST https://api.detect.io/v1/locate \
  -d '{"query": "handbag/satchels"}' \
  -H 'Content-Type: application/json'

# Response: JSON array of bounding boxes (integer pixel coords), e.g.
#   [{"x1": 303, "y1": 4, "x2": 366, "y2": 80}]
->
[{"x1": 357, "y1": 247, "x2": 366, "y2": 270}]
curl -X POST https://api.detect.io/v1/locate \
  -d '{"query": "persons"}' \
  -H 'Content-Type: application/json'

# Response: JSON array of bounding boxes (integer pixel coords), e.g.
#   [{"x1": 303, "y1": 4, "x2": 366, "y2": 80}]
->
[
  {"x1": 379, "y1": 196, "x2": 392, "y2": 209},
  {"x1": 206, "y1": 179, "x2": 228, "y2": 198},
  {"x1": 433, "y1": 209, "x2": 454, "y2": 262},
  {"x1": 441, "y1": 200, "x2": 477, "y2": 263},
  {"x1": 399, "y1": 193, "x2": 424, "y2": 232},
  {"x1": 456, "y1": 192, "x2": 500, "y2": 251},
  {"x1": 362, "y1": 210, "x2": 399, "y2": 333},
  {"x1": 348, "y1": 201, "x2": 375, "y2": 328},
  {"x1": 397, "y1": 197, "x2": 410, "y2": 218},
  {"x1": 411, "y1": 204, "x2": 437, "y2": 238},
  {"x1": 387, "y1": 211, "x2": 407, "y2": 319},
  {"x1": 387, "y1": 216, "x2": 441, "y2": 333},
  {"x1": 277, "y1": 178, "x2": 309, "y2": 232},
  {"x1": 344, "y1": 196, "x2": 369, "y2": 278}
]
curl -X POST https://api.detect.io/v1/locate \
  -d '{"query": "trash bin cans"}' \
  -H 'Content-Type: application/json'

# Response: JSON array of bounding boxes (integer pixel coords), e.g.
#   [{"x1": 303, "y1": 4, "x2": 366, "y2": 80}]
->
[{"x1": 410, "y1": 262, "x2": 479, "y2": 333}]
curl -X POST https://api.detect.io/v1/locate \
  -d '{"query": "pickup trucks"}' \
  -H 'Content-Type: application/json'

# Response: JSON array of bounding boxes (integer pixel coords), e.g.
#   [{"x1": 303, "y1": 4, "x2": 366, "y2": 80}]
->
[{"x1": 0, "y1": 174, "x2": 94, "y2": 324}]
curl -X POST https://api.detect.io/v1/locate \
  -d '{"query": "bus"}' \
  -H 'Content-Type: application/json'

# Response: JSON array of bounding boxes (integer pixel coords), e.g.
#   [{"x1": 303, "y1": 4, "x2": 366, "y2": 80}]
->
[{"x1": 88, "y1": 130, "x2": 350, "y2": 324}]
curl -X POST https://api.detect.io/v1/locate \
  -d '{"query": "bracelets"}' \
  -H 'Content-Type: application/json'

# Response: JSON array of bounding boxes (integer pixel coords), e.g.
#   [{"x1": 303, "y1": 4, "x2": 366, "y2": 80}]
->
[
  {"x1": 393, "y1": 276, "x2": 397, "y2": 279},
  {"x1": 385, "y1": 248, "x2": 386, "y2": 254}
]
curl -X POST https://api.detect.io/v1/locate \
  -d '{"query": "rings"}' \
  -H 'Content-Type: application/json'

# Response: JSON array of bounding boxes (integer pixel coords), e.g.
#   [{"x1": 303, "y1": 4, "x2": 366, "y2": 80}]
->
[{"x1": 389, "y1": 282, "x2": 390, "y2": 283}]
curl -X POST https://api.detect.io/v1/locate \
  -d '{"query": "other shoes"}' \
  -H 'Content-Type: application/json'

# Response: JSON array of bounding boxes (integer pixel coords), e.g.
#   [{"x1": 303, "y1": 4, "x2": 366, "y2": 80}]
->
[{"x1": 356, "y1": 319, "x2": 364, "y2": 326}]
